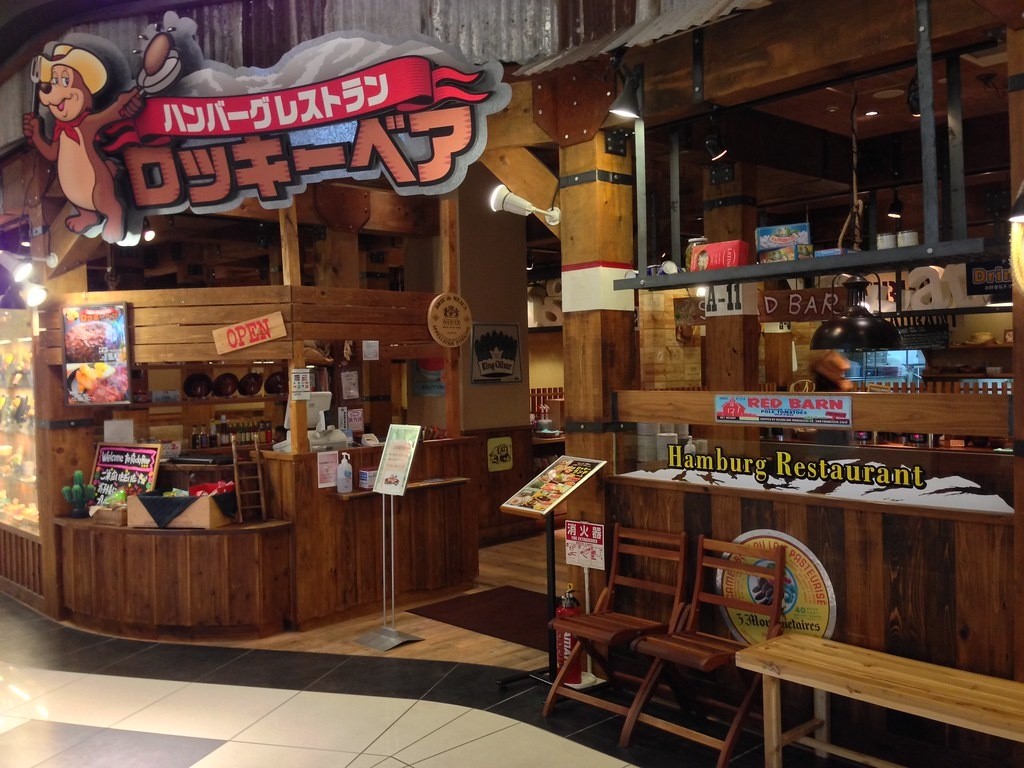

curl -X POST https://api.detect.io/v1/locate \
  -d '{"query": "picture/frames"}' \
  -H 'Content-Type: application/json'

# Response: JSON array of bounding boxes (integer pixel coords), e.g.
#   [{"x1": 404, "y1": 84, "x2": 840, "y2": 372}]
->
[
  {"x1": 84, "y1": 442, "x2": 161, "y2": 509},
  {"x1": 1004, "y1": 329, "x2": 1013, "y2": 343}
]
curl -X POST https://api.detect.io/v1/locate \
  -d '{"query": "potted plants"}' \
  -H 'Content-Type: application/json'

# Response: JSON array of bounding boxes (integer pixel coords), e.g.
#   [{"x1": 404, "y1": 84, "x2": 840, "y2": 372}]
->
[{"x1": 61, "y1": 470, "x2": 94, "y2": 518}]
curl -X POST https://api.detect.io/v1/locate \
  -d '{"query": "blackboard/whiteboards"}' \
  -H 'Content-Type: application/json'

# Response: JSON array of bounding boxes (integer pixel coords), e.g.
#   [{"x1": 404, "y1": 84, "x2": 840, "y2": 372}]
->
[{"x1": 85, "y1": 441, "x2": 163, "y2": 510}]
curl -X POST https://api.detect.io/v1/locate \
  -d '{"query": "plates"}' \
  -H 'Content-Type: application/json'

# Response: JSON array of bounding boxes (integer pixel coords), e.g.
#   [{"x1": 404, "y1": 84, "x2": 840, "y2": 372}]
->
[
  {"x1": 636, "y1": 423, "x2": 708, "y2": 462},
  {"x1": 67, "y1": 365, "x2": 89, "y2": 404}
]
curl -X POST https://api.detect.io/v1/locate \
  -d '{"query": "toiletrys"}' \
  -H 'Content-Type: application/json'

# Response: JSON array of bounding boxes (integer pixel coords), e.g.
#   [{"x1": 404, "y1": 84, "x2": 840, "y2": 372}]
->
[{"x1": 336, "y1": 452, "x2": 353, "y2": 494}]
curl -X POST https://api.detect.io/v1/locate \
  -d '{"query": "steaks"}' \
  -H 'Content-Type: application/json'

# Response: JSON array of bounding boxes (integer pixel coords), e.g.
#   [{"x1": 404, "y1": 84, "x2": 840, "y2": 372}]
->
[{"x1": 92, "y1": 366, "x2": 128, "y2": 404}]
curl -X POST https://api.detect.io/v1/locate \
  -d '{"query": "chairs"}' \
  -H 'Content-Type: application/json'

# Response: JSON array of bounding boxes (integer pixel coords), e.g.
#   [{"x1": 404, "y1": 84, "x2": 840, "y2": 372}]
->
[
  {"x1": 542, "y1": 523, "x2": 692, "y2": 747},
  {"x1": 624, "y1": 534, "x2": 787, "y2": 768}
]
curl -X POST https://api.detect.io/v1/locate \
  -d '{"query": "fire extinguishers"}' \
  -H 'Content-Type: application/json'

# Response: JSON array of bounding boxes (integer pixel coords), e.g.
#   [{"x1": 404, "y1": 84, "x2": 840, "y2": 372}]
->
[{"x1": 555, "y1": 583, "x2": 582, "y2": 684}]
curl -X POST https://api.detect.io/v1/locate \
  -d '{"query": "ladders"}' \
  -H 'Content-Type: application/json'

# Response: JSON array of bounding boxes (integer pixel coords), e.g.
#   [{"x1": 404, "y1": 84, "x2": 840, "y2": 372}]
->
[{"x1": 230, "y1": 434, "x2": 268, "y2": 524}]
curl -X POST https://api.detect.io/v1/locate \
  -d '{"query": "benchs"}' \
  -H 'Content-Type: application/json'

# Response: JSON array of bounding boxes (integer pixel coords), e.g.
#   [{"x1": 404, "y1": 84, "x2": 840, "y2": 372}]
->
[{"x1": 736, "y1": 632, "x2": 1024, "y2": 768}]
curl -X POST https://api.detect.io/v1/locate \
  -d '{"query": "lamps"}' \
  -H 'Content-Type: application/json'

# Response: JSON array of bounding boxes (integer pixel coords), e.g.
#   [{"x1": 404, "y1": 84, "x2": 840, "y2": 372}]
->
[
  {"x1": 490, "y1": 184, "x2": 561, "y2": 225},
  {"x1": 809, "y1": 90, "x2": 901, "y2": 351},
  {"x1": 907, "y1": 72, "x2": 921, "y2": 117},
  {"x1": 888, "y1": 200, "x2": 904, "y2": 219},
  {"x1": 0, "y1": 250, "x2": 59, "y2": 282},
  {"x1": 704, "y1": 135, "x2": 728, "y2": 163},
  {"x1": 0, "y1": 280, "x2": 48, "y2": 308}
]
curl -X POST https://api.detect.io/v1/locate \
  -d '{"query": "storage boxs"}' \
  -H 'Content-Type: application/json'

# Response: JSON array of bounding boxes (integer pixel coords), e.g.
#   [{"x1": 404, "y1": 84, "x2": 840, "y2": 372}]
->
[
  {"x1": 755, "y1": 223, "x2": 810, "y2": 253},
  {"x1": 127, "y1": 488, "x2": 239, "y2": 529},
  {"x1": 759, "y1": 244, "x2": 813, "y2": 264},
  {"x1": 92, "y1": 508, "x2": 127, "y2": 526},
  {"x1": 690, "y1": 240, "x2": 749, "y2": 272},
  {"x1": 358, "y1": 466, "x2": 378, "y2": 490}
]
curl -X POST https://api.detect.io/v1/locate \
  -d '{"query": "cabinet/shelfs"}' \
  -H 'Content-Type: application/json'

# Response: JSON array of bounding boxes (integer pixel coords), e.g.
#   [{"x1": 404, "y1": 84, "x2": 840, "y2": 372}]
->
[
  {"x1": 921, "y1": 343, "x2": 1013, "y2": 377},
  {"x1": 105, "y1": 393, "x2": 289, "y2": 458}
]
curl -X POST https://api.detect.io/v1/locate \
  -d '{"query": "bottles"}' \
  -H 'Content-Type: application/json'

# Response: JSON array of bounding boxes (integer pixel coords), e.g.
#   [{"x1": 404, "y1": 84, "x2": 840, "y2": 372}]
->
[
  {"x1": 199, "y1": 425, "x2": 208, "y2": 449},
  {"x1": 209, "y1": 415, "x2": 272, "y2": 448},
  {"x1": 191, "y1": 425, "x2": 199, "y2": 448}
]
